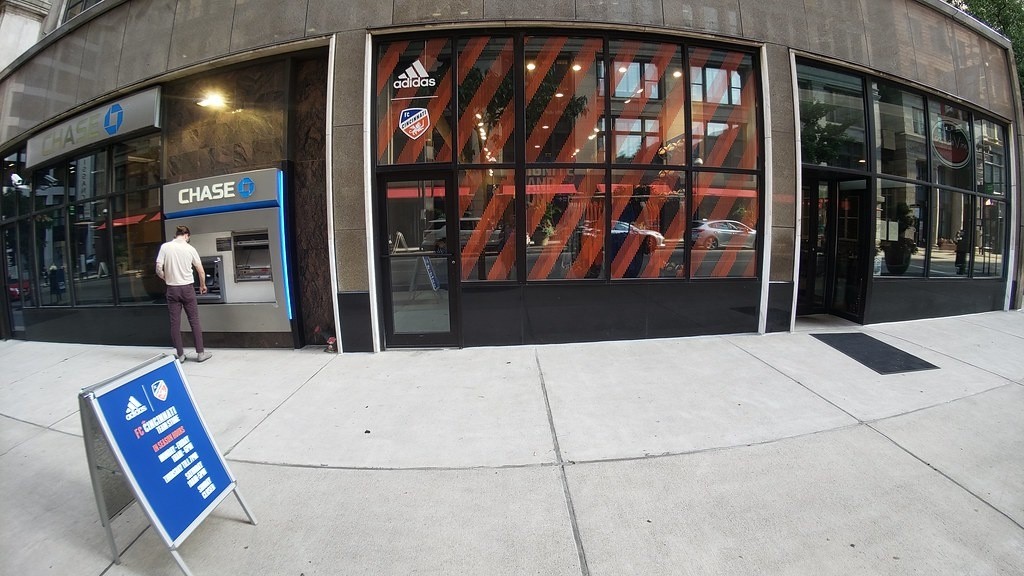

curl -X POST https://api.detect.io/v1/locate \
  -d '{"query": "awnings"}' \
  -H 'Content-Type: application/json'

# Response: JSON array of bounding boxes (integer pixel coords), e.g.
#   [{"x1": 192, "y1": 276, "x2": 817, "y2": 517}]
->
[
  {"x1": 387, "y1": 187, "x2": 472, "y2": 199},
  {"x1": 96, "y1": 212, "x2": 160, "y2": 231},
  {"x1": 495, "y1": 184, "x2": 576, "y2": 196},
  {"x1": 596, "y1": 184, "x2": 671, "y2": 196},
  {"x1": 692, "y1": 186, "x2": 758, "y2": 198}
]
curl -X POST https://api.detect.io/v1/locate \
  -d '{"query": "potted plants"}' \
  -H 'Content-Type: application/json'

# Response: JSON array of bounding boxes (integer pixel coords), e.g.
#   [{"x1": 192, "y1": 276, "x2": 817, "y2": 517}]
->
[{"x1": 878, "y1": 203, "x2": 921, "y2": 274}]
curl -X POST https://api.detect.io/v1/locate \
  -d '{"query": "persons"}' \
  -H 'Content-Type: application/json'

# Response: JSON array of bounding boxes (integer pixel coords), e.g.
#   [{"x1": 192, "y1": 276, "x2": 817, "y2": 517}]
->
[
  {"x1": 156, "y1": 225, "x2": 212, "y2": 363},
  {"x1": 955, "y1": 229, "x2": 968, "y2": 275}
]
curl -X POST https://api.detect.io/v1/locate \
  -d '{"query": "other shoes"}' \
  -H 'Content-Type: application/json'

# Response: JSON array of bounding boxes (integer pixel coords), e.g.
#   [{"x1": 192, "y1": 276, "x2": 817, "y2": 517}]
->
[
  {"x1": 198, "y1": 352, "x2": 212, "y2": 362},
  {"x1": 179, "y1": 355, "x2": 187, "y2": 363}
]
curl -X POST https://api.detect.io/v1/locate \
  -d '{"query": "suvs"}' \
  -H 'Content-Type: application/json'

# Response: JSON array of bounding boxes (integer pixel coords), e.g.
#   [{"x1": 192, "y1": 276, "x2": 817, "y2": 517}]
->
[{"x1": 422, "y1": 218, "x2": 531, "y2": 254}]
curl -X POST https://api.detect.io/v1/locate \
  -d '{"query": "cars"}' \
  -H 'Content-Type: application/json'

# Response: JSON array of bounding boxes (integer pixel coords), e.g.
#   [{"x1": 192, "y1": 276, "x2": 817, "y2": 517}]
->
[
  {"x1": 8, "y1": 277, "x2": 33, "y2": 300},
  {"x1": 568, "y1": 220, "x2": 667, "y2": 254},
  {"x1": 685, "y1": 218, "x2": 756, "y2": 250}
]
